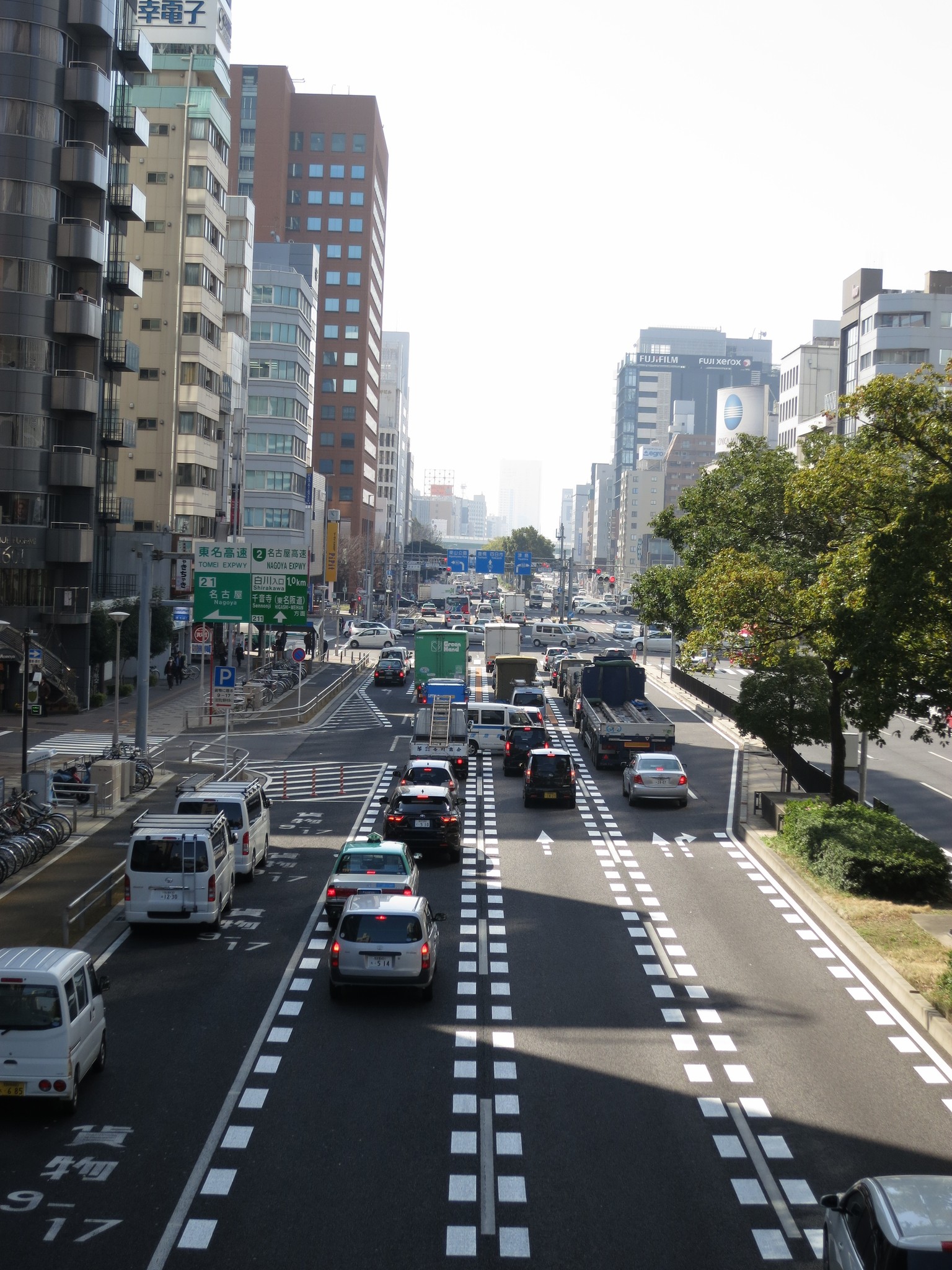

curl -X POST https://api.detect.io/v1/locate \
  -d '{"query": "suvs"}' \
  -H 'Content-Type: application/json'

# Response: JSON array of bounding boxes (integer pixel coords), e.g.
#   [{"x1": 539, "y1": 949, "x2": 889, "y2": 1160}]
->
[
  {"x1": 379, "y1": 784, "x2": 468, "y2": 864},
  {"x1": 328, "y1": 894, "x2": 448, "y2": 1002}
]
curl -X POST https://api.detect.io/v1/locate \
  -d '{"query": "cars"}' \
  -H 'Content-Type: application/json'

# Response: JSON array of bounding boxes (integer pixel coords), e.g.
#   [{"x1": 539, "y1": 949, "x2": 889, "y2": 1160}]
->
[
  {"x1": 819, "y1": 1175, "x2": 952, "y2": 1270},
  {"x1": 323, "y1": 832, "x2": 424, "y2": 928},
  {"x1": 340, "y1": 573, "x2": 789, "y2": 809}
]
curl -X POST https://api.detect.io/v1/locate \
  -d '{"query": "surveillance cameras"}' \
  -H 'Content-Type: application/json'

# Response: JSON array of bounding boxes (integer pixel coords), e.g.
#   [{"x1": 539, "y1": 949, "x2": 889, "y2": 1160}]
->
[{"x1": 32, "y1": 672, "x2": 42, "y2": 686}]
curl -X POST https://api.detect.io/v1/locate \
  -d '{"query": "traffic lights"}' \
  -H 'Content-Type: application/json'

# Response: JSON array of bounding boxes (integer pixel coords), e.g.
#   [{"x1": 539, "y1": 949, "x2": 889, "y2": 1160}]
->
[
  {"x1": 596, "y1": 575, "x2": 616, "y2": 589},
  {"x1": 535, "y1": 562, "x2": 550, "y2": 568},
  {"x1": 437, "y1": 566, "x2": 451, "y2": 576},
  {"x1": 587, "y1": 569, "x2": 600, "y2": 576}
]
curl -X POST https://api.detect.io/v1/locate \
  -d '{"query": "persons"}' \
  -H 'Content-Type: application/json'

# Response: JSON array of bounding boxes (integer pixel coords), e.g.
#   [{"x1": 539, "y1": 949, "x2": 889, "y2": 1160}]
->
[
  {"x1": 304, "y1": 599, "x2": 357, "y2": 659},
  {"x1": 164, "y1": 630, "x2": 288, "y2": 690}
]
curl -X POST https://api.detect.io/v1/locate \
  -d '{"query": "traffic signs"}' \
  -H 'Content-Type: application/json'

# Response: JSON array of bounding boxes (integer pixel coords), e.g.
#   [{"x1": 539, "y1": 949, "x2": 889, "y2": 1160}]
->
[
  {"x1": 214, "y1": 666, "x2": 236, "y2": 709},
  {"x1": 447, "y1": 549, "x2": 469, "y2": 572},
  {"x1": 514, "y1": 552, "x2": 532, "y2": 576},
  {"x1": 29, "y1": 649, "x2": 42, "y2": 665},
  {"x1": 193, "y1": 541, "x2": 308, "y2": 625},
  {"x1": 475, "y1": 550, "x2": 505, "y2": 574}
]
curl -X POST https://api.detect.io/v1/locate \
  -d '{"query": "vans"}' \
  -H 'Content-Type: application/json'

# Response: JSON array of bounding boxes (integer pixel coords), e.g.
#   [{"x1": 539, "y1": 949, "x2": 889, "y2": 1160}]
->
[
  {"x1": 123, "y1": 808, "x2": 239, "y2": 933},
  {"x1": 225, "y1": 623, "x2": 276, "y2": 652},
  {"x1": 0, "y1": 945, "x2": 111, "y2": 1115},
  {"x1": 173, "y1": 778, "x2": 274, "y2": 883}
]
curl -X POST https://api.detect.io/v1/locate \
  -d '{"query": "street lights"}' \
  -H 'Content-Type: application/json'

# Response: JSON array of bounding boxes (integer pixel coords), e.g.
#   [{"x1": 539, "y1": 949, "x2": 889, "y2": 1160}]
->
[
  {"x1": 321, "y1": 474, "x2": 335, "y2": 615},
  {"x1": 107, "y1": 610, "x2": 131, "y2": 749},
  {"x1": 363, "y1": 494, "x2": 375, "y2": 620},
  {"x1": 317, "y1": 585, "x2": 329, "y2": 652}
]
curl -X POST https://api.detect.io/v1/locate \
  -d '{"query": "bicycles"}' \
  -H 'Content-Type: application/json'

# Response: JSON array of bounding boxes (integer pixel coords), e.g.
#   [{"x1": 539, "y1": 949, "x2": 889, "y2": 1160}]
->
[
  {"x1": 149, "y1": 665, "x2": 160, "y2": 681},
  {"x1": 182, "y1": 663, "x2": 200, "y2": 680},
  {"x1": 0, "y1": 739, "x2": 155, "y2": 883},
  {"x1": 215, "y1": 656, "x2": 308, "y2": 718}
]
